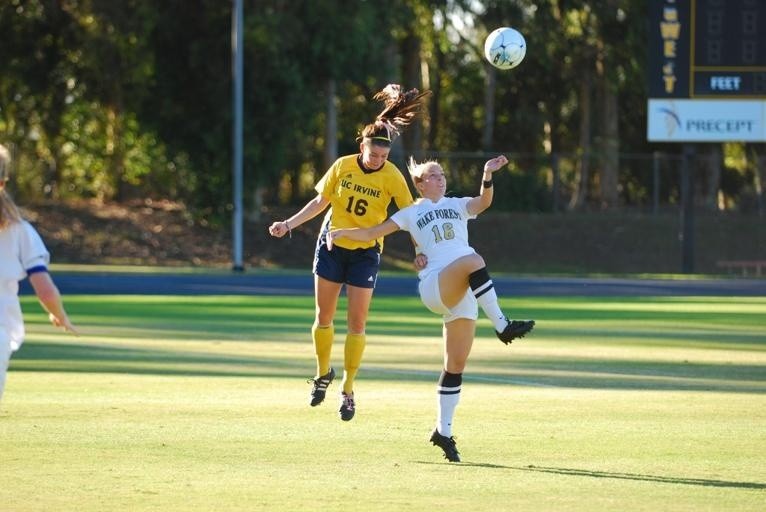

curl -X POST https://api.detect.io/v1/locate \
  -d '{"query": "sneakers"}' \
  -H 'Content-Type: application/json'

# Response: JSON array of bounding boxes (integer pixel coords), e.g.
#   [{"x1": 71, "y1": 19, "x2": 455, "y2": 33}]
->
[
  {"x1": 429, "y1": 427, "x2": 461, "y2": 462},
  {"x1": 309, "y1": 367, "x2": 335, "y2": 407},
  {"x1": 339, "y1": 388, "x2": 356, "y2": 421},
  {"x1": 495, "y1": 319, "x2": 535, "y2": 345}
]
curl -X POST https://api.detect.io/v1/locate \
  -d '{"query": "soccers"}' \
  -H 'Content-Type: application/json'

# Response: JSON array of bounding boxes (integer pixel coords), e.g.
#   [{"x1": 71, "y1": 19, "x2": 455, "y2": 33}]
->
[{"x1": 485, "y1": 27, "x2": 527, "y2": 70}]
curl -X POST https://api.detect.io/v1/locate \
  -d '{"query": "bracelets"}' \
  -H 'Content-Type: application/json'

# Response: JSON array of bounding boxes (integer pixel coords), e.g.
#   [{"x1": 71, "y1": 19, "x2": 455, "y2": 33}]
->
[
  {"x1": 284, "y1": 221, "x2": 291, "y2": 240},
  {"x1": 482, "y1": 180, "x2": 496, "y2": 188},
  {"x1": 415, "y1": 247, "x2": 421, "y2": 256}
]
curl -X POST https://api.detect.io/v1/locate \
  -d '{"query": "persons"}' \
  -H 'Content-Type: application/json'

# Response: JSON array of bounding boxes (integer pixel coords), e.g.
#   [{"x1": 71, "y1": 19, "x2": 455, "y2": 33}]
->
[
  {"x1": 325, "y1": 155, "x2": 534, "y2": 462},
  {"x1": 0, "y1": 144, "x2": 81, "y2": 399},
  {"x1": 269, "y1": 84, "x2": 428, "y2": 421}
]
